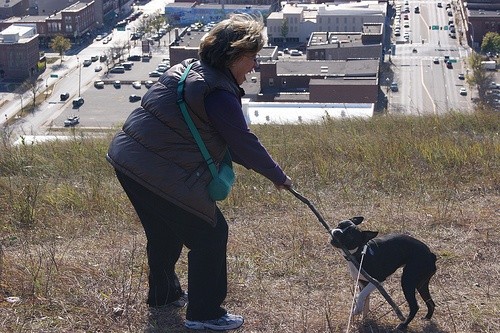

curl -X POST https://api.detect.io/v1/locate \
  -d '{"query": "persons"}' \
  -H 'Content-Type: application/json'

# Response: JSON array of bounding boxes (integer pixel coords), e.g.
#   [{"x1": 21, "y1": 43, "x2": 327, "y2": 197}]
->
[{"x1": 106, "y1": 11, "x2": 292, "y2": 330}]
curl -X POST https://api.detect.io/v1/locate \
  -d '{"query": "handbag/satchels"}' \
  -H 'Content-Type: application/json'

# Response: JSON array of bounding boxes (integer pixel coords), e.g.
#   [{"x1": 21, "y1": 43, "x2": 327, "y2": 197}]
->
[{"x1": 208, "y1": 147, "x2": 235, "y2": 201}]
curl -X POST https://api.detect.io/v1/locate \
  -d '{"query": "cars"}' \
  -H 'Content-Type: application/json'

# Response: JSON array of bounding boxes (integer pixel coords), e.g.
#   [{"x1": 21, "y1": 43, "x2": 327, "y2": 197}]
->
[
  {"x1": 390, "y1": 0, "x2": 468, "y2": 95},
  {"x1": 59, "y1": 9, "x2": 303, "y2": 127}
]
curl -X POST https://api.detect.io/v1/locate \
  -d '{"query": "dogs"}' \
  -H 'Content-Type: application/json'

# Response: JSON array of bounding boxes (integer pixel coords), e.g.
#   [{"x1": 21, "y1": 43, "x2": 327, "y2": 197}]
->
[{"x1": 328, "y1": 216, "x2": 437, "y2": 331}]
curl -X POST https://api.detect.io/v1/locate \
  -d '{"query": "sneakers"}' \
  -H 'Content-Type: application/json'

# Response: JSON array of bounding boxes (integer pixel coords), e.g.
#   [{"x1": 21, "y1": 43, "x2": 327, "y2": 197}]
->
[
  {"x1": 152, "y1": 289, "x2": 189, "y2": 307},
  {"x1": 185, "y1": 312, "x2": 245, "y2": 330}
]
